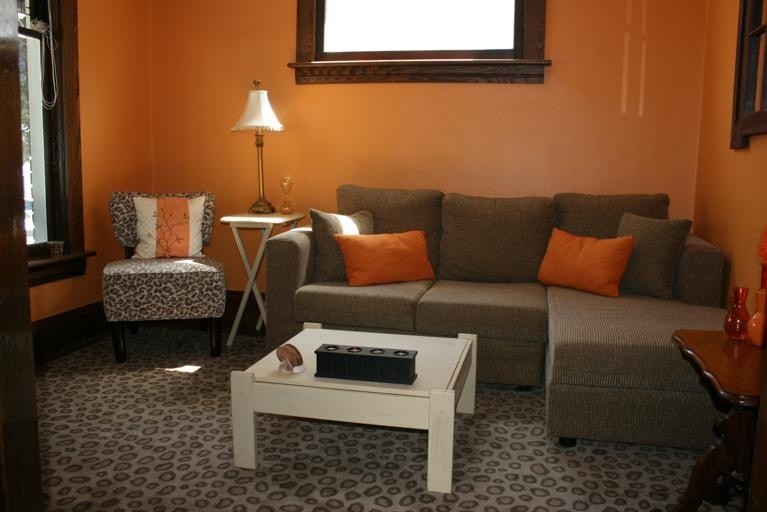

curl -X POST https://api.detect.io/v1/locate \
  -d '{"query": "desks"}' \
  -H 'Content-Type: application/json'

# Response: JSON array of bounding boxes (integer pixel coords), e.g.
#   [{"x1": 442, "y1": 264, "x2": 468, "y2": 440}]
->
[
  {"x1": 669, "y1": 328, "x2": 767, "y2": 512},
  {"x1": 219, "y1": 209, "x2": 307, "y2": 350}
]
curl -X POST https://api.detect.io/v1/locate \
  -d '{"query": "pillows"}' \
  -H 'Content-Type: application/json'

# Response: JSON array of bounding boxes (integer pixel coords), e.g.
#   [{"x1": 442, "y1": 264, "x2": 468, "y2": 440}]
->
[
  {"x1": 551, "y1": 190, "x2": 672, "y2": 240},
  {"x1": 439, "y1": 191, "x2": 555, "y2": 284},
  {"x1": 308, "y1": 206, "x2": 375, "y2": 284},
  {"x1": 332, "y1": 227, "x2": 438, "y2": 288},
  {"x1": 130, "y1": 194, "x2": 210, "y2": 261},
  {"x1": 537, "y1": 226, "x2": 636, "y2": 300},
  {"x1": 616, "y1": 210, "x2": 694, "y2": 301},
  {"x1": 333, "y1": 184, "x2": 443, "y2": 280}
]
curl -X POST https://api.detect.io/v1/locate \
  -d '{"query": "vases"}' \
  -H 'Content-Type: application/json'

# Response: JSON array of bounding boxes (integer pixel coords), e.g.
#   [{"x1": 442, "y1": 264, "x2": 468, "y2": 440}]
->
[
  {"x1": 747, "y1": 288, "x2": 767, "y2": 346},
  {"x1": 723, "y1": 286, "x2": 752, "y2": 341}
]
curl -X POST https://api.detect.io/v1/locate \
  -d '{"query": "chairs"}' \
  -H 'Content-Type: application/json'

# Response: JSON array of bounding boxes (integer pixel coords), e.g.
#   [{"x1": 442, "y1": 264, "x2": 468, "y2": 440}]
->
[{"x1": 97, "y1": 189, "x2": 228, "y2": 364}]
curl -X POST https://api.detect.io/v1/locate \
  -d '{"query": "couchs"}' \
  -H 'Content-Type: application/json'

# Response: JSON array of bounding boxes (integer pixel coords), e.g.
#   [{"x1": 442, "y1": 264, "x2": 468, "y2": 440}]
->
[{"x1": 262, "y1": 182, "x2": 735, "y2": 452}]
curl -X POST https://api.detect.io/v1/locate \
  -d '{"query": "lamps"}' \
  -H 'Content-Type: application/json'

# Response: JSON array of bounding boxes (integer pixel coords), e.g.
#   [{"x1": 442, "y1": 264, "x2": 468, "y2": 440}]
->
[{"x1": 230, "y1": 77, "x2": 287, "y2": 216}]
